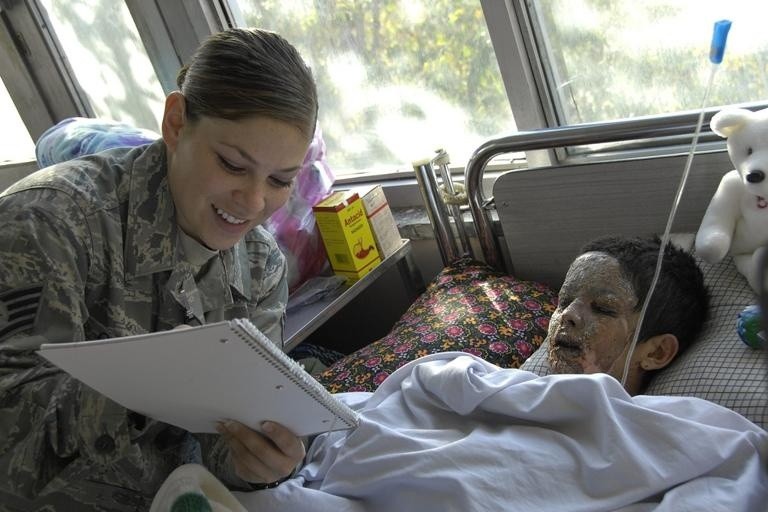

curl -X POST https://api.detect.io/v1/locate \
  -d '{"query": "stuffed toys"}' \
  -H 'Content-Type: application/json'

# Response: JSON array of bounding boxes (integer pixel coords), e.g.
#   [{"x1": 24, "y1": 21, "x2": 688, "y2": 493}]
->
[{"x1": 697, "y1": 106, "x2": 768, "y2": 296}]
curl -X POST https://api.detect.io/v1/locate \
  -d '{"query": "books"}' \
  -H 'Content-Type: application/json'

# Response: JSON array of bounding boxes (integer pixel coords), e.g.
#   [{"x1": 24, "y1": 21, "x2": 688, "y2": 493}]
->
[{"x1": 36, "y1": 319, "x2": 362, "y2": 441}]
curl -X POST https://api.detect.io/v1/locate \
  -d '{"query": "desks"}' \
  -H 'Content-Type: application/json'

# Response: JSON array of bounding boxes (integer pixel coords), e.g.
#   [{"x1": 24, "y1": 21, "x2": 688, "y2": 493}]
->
[{"x1": 280, "y1": 236, "x2": 428, "y2": 356}]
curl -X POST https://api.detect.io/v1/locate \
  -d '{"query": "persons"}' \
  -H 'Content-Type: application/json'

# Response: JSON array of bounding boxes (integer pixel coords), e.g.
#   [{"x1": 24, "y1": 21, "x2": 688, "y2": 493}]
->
[
  {"x1": 1, "y1": 29, "x2": 318, "y2": 512},
  {"x1": 238, "y1": 235, "x2": 768, "y2": 512}
]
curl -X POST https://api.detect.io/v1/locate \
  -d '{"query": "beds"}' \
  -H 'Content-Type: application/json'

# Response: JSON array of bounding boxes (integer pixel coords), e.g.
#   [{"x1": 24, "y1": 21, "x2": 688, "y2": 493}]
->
[{"x1": 313, "y1": 104, "x2": 768, "y2": 512}]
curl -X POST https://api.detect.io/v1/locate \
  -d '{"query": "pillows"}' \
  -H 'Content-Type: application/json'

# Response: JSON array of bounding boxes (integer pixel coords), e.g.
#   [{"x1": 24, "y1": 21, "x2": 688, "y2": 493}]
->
[
  {"x1": 313, "y1": 257, "x2": 557, "y2": 391},
  {"x1": 516, "y1": 231, "x2": 768, "y2": 425}
]
